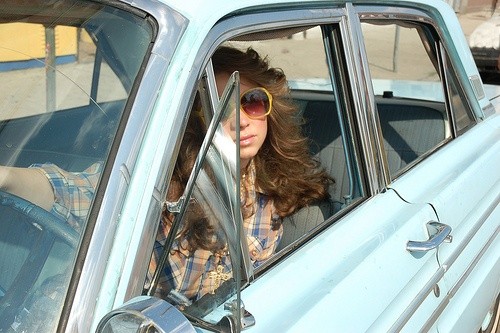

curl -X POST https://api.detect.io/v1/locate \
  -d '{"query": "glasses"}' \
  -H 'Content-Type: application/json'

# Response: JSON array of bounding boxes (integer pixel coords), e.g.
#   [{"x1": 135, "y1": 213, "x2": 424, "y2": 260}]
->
[{"x1": 240, "y1": 87, "x2": 272, "y2": 119}]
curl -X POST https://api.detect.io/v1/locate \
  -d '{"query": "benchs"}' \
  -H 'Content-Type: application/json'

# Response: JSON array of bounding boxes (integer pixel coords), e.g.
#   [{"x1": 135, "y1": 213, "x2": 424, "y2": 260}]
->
[{"x1": 290, "y1": 91, "x2": 445, "y2": 202}]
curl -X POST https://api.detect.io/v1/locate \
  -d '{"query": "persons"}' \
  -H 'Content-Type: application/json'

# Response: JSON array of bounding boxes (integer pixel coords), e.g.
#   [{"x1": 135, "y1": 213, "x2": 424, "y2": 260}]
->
[{"x1": 0, "y1": 44, "x2": 338, "y2": 332}]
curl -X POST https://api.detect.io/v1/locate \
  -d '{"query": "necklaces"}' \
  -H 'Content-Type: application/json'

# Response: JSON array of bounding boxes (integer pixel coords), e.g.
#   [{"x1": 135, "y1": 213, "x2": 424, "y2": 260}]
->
[{"x1": 239, "y1": 168, "x2": 246, "y2": 206}]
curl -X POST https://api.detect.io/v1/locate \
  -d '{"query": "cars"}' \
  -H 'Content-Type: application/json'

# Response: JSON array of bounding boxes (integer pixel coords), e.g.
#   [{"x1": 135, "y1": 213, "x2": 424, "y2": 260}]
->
[{"x1": 0, "y1": 0, "x2": 498, "y2": 333}]
